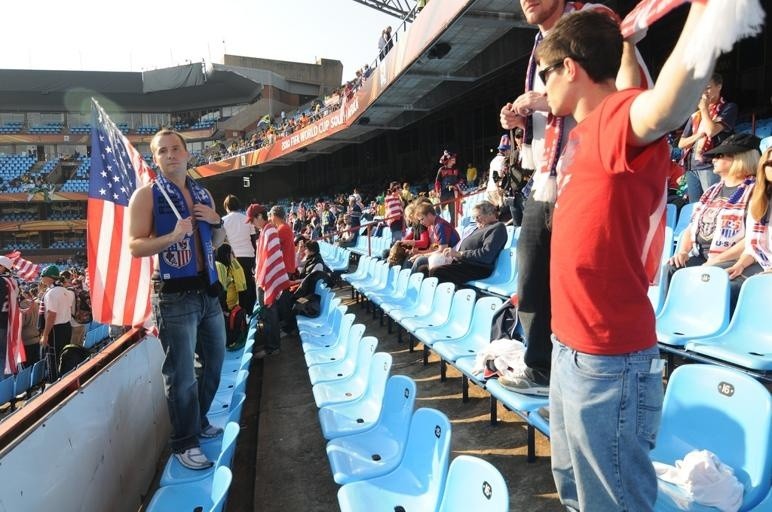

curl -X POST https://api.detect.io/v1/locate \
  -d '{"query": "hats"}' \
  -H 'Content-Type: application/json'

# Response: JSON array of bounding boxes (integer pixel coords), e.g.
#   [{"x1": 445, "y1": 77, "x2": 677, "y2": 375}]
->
[
  {"x1": 514, "y1": 128, "x2": 524, "y2": 137},
  {"x1": 40, "y1": 265, "x2": 61, "y2": 279},
  {"x1": 243, "y1": 203, "x2": 264, "y2": 225},
  {"x1": 439, "y1": 150, "x2": 456, "y2": 163},
  {"x1": 701, "y1": 134, "x2": 763, "y2": 156},
  {"x1": 498, "y1": 134, "x2": 513, "y2": 149},
  {"x1": 0, "y1": 256, "x2": 13, "y2": 272}
]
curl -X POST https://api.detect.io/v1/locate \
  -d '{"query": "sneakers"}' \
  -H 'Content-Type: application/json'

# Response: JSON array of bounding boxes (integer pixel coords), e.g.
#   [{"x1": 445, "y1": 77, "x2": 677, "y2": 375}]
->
[
  {"x1": 199, "y1": 423, "x2": 224, "y2": 437},
  {"x1": 175, "y1": 446, "x2": 212, "y2": 470},
  {"x1": 280, "y1": 328, "x2": 299, "y2": 338},
  {"x1": 496, "y1": 371, "x2": 550, "y2": 397},
  {"x1": 253, "y1": 347, "x2": 280, "y2": 359}
]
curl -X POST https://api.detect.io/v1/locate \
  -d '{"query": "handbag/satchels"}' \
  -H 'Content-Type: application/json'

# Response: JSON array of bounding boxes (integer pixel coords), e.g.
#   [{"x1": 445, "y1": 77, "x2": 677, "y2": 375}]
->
[
  {"x1": 387, "y1": 241, "x2": 412, "y2": 266},
  {"x1": 58, "y1": 343, "x2": 98, "y2": 383},
  {"x1": 290, "y1": 294, "x2": 321, "y2": 316}
]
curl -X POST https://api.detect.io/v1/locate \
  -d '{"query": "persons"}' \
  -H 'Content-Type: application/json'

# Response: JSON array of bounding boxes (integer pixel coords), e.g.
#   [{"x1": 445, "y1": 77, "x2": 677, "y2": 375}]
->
[
  {"x1": 0, "y1": 0, "x2": 771, "y2": 375},
  {"x1": 532, "y1": 1, "x2": 723, "y2": 512},
  {"x1": 126, "y1": 126, "x2": 225, "y2": 472},
  {"x1": 499, "y1": 0, "x2": 625, "y2": 399}
]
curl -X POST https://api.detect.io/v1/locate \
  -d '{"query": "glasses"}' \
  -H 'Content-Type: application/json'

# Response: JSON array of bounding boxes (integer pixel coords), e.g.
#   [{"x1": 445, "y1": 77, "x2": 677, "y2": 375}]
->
[{"x1": 539, "y1": 62, "x2": 562, "y2": 86}]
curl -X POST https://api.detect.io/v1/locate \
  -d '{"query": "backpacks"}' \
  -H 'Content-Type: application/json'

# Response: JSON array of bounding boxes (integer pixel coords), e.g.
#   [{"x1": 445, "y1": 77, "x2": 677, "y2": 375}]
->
[
  {"x1": 68, "y1": 287, "x2": 92, "y2": 324},
  {"x1": 226, "y1": 304, "x2": 246, "y2": 351}
]
